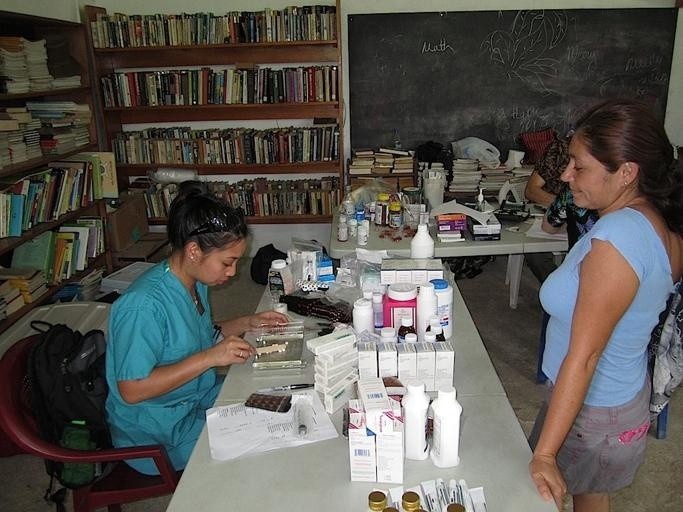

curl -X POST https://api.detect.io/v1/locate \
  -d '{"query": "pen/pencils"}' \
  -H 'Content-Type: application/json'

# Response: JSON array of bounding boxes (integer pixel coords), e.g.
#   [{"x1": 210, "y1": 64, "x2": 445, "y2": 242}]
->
[{"x1": 257, "y1": 384, "x2": 314, "y2": 394}]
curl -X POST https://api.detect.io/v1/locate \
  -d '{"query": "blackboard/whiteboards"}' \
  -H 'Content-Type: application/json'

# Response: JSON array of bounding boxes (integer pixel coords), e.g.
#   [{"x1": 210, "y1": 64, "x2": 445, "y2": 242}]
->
[{"x1": 348, "y1": 7, "x2": 680, "y2": 165}]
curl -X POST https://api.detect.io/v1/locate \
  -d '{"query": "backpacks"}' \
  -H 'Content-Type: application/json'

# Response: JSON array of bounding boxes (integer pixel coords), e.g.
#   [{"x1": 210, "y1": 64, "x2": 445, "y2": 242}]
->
[{"x1": 27, "y1": 315, "x2": 114, "y2": 488}]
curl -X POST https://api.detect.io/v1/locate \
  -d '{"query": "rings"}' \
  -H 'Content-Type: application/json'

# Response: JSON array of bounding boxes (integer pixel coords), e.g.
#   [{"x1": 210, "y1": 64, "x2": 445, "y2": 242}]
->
[{"x1": 238, "y1": 349, "x2": 243, "y2": 358}]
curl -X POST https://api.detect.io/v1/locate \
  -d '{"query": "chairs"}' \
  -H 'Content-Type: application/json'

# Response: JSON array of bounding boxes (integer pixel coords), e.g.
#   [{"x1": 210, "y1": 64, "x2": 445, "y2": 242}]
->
[
  {"x1": 539, "y1": 312, "x2": 672, "y2": 439},
  {"x1": 498, "y1": 178, "x2": 564, "y2": 310},
  {"x1": 0, "y1": 332, "x2": 183, "y2": 512}
]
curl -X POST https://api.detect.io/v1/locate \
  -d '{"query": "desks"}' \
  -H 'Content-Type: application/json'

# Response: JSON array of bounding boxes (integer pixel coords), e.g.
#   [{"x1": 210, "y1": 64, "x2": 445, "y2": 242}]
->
[{"x1": 167, "y1": 204, "x2": 569, "y2": 512}]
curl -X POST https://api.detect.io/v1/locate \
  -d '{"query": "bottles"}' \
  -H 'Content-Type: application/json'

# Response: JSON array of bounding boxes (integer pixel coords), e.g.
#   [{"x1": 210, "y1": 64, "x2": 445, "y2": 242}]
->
[
  {"x1": 266, "y1": 259, "x2": 295, "y2": 305},
  {"x1": 337, "y1": 193, "x2": 467, "y2": 512}
]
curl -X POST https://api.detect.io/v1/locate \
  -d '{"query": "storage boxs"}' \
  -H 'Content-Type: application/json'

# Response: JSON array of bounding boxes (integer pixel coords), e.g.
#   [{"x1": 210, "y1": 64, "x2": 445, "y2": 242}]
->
[
  {"x1": 85, "y1": 195, "x2": 171, "y2": 295},
  {"x1": 0, "y1": 302, "x2": 111, "y2": 365}
]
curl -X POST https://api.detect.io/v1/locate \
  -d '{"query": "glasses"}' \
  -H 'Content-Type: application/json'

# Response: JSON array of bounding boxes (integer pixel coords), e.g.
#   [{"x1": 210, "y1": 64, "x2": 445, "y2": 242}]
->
[{"x1": 186, "y1": 206, "x2": 248, "y2": 241}]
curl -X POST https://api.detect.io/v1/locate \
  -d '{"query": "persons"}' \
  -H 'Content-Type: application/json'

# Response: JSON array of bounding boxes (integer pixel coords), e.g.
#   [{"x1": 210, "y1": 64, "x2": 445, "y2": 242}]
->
[
  {"x1": 102, "y1": 180, "x2": 291, "y2": 479},
  {"x1": 539, "y1": 184, "x2": 599, "y2": 253},
  {"x1": 523, "y1": 121, "x2": 573, "y2": 283},
  {"x1": 526, "y1": 96, "x2": 683, "y2": 511}
]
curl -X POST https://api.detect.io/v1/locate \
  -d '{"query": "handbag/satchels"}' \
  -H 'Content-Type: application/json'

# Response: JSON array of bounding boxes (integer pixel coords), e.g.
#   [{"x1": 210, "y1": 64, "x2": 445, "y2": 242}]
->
[
  {"x1": 517, "y1": 128, "x2": 560, "y2": 168},
  {"x1": 646, "y1": 267, "x2": 683, "y2": 415}
]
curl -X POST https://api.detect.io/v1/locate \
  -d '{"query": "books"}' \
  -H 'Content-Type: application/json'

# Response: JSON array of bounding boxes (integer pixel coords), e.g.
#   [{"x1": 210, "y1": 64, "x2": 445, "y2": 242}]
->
[
  {"x1": 91, "y1": 5, "x2": 337, "y2": 48},
  {"x1": 346, "y1": 144, "x2": 538, "y2": 194},
  {"x1": 126, "y1": 177, "x2": 341, "y2": 217},
  {"x1": 1, "y1": 153, "x2": 118, "y2": 318},
  {"x1": 2, "y1": 36, "x2": 81, "y2": 93},
  {"x1": 111, "y1": 126, "x2": 338, "y2": 165},
  {"x1": 1, "y1": 102, "x2": 96, "y2": 165},
  {"x1": 101, "y1": 63, "x2": 339, "y2": 108}
]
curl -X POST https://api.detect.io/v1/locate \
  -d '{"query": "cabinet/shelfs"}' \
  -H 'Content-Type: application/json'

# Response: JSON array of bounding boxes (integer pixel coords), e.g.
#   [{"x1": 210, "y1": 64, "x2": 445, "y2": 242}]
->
[
  {"x1": 83, "y1": 0, "x2": 344, "y2": 224},
  {"x1": 0, "y1": 10, "x2": 109, "y2": 334}
]
